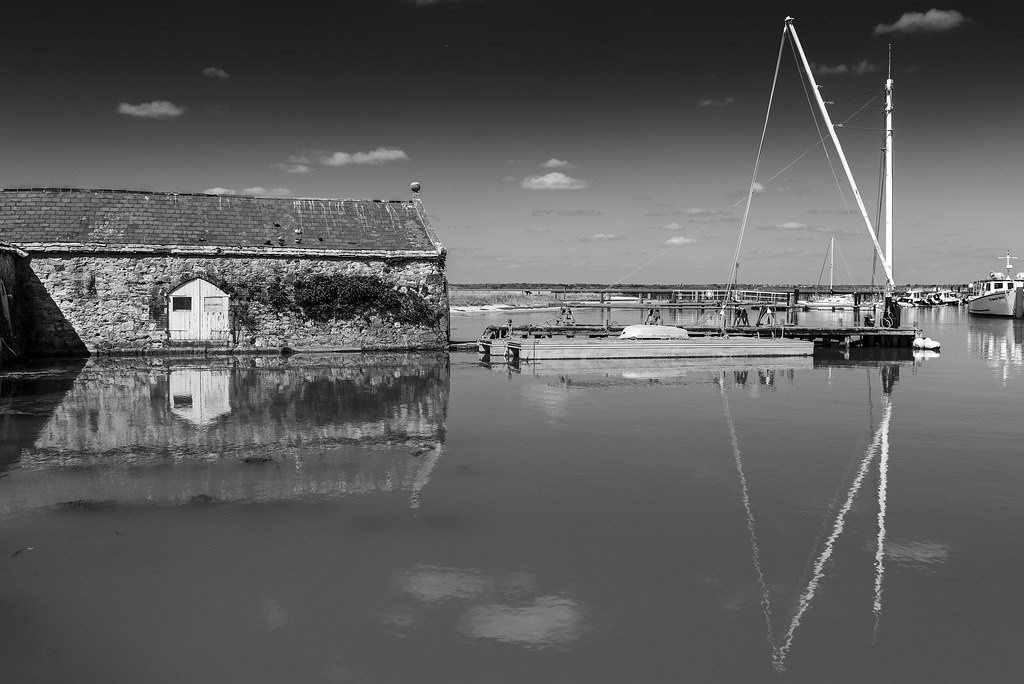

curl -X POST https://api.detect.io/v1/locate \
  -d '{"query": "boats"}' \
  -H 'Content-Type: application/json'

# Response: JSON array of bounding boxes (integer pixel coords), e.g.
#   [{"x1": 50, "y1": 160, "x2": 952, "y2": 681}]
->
[
  {"x1": 967, "y1": 250, "x2": 1024, "y2": 316},
  {"x1": 478, "y1": 323, "x2": 814, "y2": 358},
  {"x1": 804, "y1": 237, "x2": 961, "y2": 307}
]
